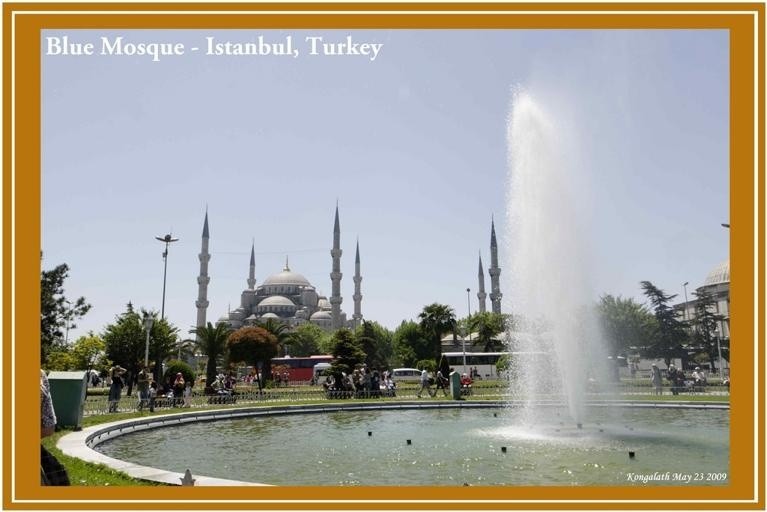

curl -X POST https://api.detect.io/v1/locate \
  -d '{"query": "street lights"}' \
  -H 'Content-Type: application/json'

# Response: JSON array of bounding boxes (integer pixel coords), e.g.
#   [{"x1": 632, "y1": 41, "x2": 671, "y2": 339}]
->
[
  {"x1": 459, "y1": 323, "x2": 467, "y2": 377},
  {"x1": 153, "y1": 234, "x2": 180, "y2": 320},
  {"x1": 143, "y1": 312, "x2": 155, "y2": 368},
  {"x1": 682, "y1": 280, "x2": 691, "y2": 351},
  {"x1": 712, "y1": 326, "x2": 723, "y2": 383},
  {"x1": 64, "y1": 300, "x2": 71, "y2": 346},
  {"x1": 466, "y1": 287, "x2": 472, "y2": 351}
]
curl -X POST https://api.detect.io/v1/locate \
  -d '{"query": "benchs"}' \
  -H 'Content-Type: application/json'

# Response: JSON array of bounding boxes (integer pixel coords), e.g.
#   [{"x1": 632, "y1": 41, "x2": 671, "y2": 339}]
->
[
  {"x1": 322, "y1": 384, "x2": 353, "y2": 399},
  {"x1": 676, "y1": 377, "x2": 708, "y2": 392},
  {"x1": 203, "y1": 387, "x2": 239, "y2": 405}
]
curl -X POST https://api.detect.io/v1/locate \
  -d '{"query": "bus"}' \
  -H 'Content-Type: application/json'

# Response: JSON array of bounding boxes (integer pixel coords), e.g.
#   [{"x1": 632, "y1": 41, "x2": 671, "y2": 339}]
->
[
  {"x1": 625, "y1": 346, "x2": 730, "y2": 371},
  {"x1": 438, "y1": 351, "x2": 553, "y2": 383},
  {"x1": 312, "y1": 361, "x2": 366, "y2": 388},
  {"x1": 214, "y1": 365, "x2": 254, "y2": 378},
  {"x1": 269, "y1": 354, "x2": 343, "y2": 385}
]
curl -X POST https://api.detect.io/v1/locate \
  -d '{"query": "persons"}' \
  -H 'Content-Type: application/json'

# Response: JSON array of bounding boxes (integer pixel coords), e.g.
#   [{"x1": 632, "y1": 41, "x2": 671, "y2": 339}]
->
[
  {"x1": 209, "y1": 365, "x2": 290, "y2": 395},
  {"x1": 649, "y1": 361, "x2": 708, "y2": 395},
  {"x1": 417, "y1": 366, "x2": 481, "y2": 397},
  {"x1": 88, "y1": 367, "x2": 193, "y2": 412},
  {"x1": 322, "y1": 369, "x2": 396, "y2": 398},
  {"x1": 40, "y1": 370, "x2": 57, "y2": 439}
]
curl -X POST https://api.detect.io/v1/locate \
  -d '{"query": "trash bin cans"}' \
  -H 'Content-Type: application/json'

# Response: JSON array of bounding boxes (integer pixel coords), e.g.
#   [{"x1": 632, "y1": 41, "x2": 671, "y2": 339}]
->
[{"x1": 47, "y1": 370, "x2": 88, "y2": 431}]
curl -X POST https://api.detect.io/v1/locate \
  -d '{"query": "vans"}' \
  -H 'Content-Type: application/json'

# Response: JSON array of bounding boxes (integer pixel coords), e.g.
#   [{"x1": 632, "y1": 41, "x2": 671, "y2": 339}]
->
[
  {"x1": 607, "y1": 356, "x2": 628, "y2": 371},
  {"x1": 389, "y1": 367, "x2": 426, "y2": 384}
]
